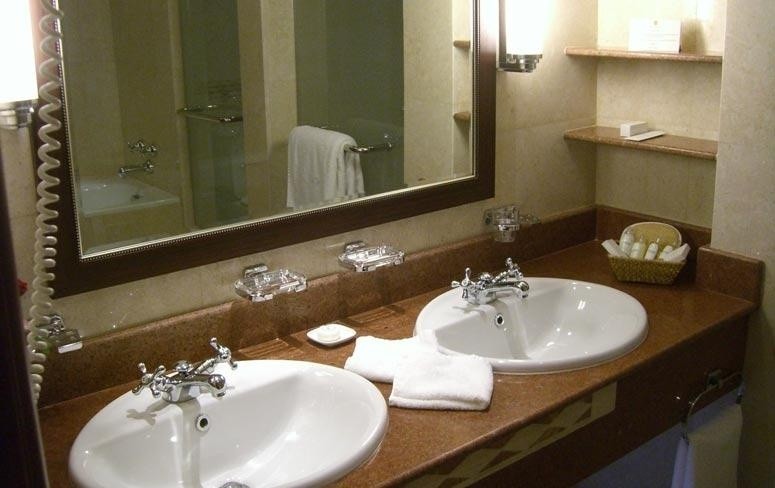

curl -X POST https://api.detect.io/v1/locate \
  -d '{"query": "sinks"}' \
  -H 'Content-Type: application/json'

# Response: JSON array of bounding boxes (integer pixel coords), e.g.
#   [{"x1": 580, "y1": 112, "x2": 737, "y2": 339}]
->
[
  {"x1": 67, "y1": 357, "x2": 390, "y2": 488},
  {"x1": 411, "y1": 276, "x2": 651, "y2": 377}
]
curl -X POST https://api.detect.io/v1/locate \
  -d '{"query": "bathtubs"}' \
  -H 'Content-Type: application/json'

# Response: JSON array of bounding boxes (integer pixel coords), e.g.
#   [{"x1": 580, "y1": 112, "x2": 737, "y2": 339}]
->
[{"x1": 79, "y1": 173, "x2": 180, "y2": 218}]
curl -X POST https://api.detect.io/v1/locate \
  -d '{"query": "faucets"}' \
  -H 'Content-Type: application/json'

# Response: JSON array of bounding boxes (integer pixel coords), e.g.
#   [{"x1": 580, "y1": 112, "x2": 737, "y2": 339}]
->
[
  {"x1": 117, "y1": 159, "x2": 154, "y2": 176},
  {"x1": 162, "y1": 358, "x2": 228, "y2": 403},
  {"x1": 470, "y1": 270, "x2": 531, "y2": 308}
]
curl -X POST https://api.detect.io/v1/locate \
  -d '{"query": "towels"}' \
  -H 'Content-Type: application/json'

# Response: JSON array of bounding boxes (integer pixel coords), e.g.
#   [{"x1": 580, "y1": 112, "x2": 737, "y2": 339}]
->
[
  {"x1": 343, "y1": 335, "x2": 494, "y2": 416},
  {"x1": 282, "y1": 120, "x2": 365, "y2": 211},
  {"x1": 671, "y1": 404, "x2": 746, "y2": 488}
]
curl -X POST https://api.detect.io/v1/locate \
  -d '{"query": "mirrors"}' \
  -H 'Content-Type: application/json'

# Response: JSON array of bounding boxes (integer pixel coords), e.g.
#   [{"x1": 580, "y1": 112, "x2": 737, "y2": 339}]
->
[{"x1": 28, "y1": 0, "x2": 499, "y2": 292}]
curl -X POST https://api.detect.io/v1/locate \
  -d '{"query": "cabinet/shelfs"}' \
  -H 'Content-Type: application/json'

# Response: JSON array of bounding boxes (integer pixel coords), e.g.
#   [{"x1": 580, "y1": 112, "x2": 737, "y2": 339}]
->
[{"x1": 563, "y1": 46, "x2": 723, "y2": 163}]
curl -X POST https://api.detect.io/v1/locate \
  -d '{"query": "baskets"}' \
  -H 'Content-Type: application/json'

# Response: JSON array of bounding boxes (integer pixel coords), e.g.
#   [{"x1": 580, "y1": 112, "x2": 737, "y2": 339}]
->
[{"x1": 605, "y1": 222, "x2": 687, "y2": 285}]
